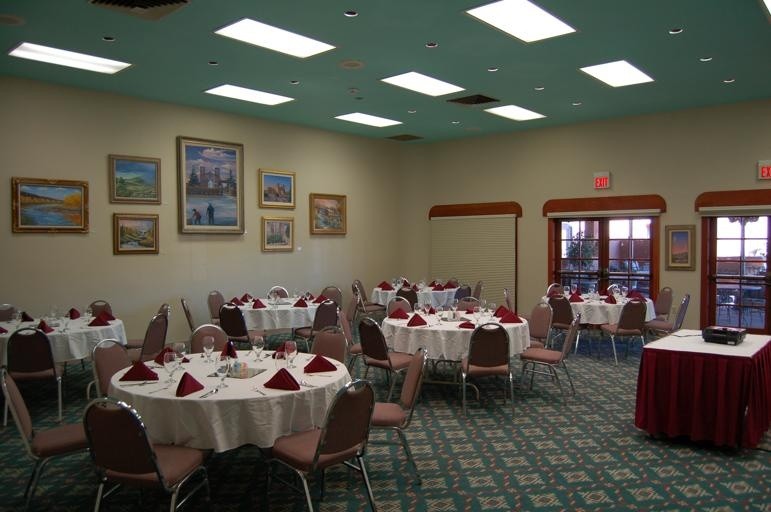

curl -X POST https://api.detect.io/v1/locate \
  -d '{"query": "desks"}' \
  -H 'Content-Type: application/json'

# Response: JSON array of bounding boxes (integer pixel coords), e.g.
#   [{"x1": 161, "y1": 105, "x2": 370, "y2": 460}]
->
[
  {"x1": 636, "y1": 327, "x2": 771, "y2": 455},
  {"x1": 383, "y1": 306, "x2": 530, "y2": 401},
  {"x1": 371, "y1": 286, "x2": 462, "y2": 305}
]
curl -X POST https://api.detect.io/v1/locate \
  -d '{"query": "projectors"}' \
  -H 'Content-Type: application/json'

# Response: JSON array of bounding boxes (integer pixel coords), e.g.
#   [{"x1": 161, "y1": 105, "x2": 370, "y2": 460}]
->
[{"x1": 702, "y1": 326, "x2": 747, "y2": 345}]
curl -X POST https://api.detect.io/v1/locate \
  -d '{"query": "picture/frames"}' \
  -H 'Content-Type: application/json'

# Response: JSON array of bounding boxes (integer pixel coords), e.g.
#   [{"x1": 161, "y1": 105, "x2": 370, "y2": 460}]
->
[
  {"x1": 664, "y1": 222, "x2": 698, "y2": 274},
  {"x1": 106, "y1": 152, "x2": 164, "y2": 208},
  {"x1": 10, "y1": 174, "x2": 91, "y2": 238},
  {"x1": 307, "y1": 192, "x2": 349, "y2": 238},
  {"x1": 111, "y1": 212, "x2": 161, "y2": 257},
  {"x1": 175, "y1": 133, "x2": 248, "y2": 236},
  {"x1": 260, "y1": 217, "x2": 299, "y2": 254},
  {"x1": 257, "y1": 167, "x2": 298, "y2": 211}
]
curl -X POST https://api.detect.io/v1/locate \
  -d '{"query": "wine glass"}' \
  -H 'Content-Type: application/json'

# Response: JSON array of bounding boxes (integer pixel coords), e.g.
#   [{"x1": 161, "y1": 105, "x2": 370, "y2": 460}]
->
[
  {"x1": 246, "y1": 277, "x2": 632, "y2": 332},
  {"x1": 1, "y1": 305, "x2": 94, "y2": 333},
  {"x1": 161, "y1": 334, "x2": 297, "y2": 389}
]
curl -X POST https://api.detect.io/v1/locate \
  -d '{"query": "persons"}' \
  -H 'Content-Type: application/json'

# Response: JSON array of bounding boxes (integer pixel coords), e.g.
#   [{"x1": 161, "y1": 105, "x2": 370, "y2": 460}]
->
[
  {"x1": 191, "y1": 208, "x2": 201, "y2": 225},
  {"x1": 206, "y1": 201, "x2": 214, "y2": 225}
]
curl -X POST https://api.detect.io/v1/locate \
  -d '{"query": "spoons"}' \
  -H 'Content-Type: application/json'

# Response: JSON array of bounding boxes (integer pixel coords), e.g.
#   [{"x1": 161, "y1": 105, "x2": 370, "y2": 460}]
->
[{"x1": 118, "y1": 356, "x2": 341, "y2": 399}]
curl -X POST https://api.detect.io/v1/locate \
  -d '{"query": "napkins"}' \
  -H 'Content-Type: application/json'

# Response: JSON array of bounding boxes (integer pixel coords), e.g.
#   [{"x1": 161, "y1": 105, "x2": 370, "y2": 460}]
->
[{"x1": 430, "y1": 279, "x2": 459, "y2": 293}]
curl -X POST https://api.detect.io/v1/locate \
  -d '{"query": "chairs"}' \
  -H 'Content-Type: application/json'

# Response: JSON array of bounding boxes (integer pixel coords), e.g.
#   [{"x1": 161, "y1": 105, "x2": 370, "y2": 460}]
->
[
  {"x1": 454, "y1": 280, "x2": 592, "y2": 419},
  {"x1": 0, "y1": 279, "x2": 429, "y2": 512},
  {"x1": 597, "y1": 284, "x2": 691, "y2": 367}
]
